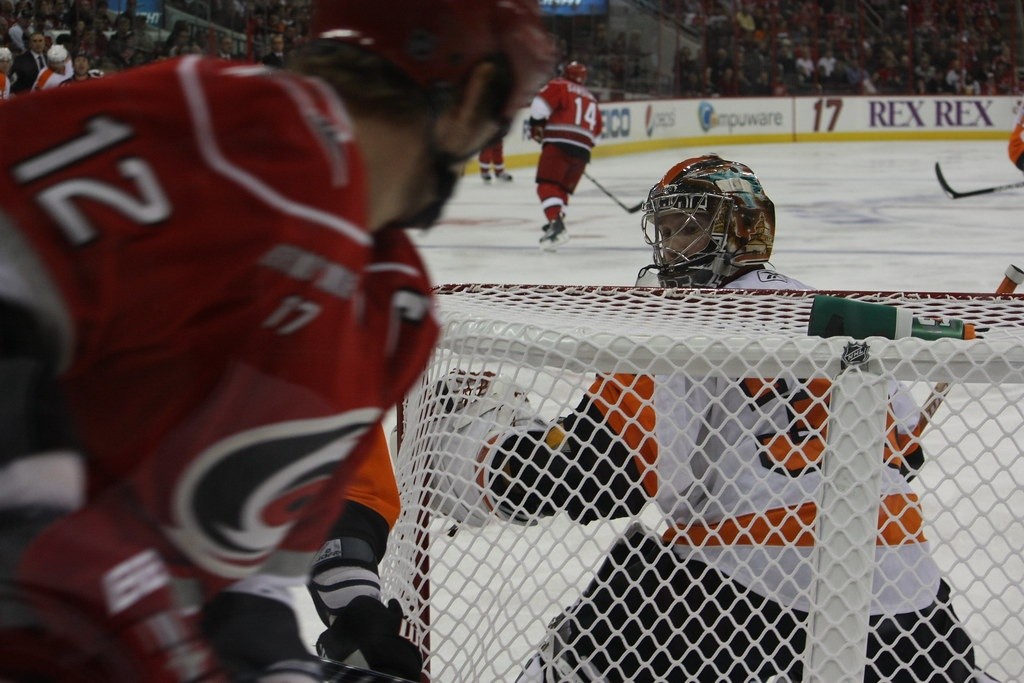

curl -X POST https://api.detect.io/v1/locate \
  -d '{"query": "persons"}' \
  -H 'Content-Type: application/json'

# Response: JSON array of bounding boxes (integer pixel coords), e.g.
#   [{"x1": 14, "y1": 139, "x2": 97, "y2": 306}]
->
[
  {"x1": 393, "y1": 154, "x2": 975, "y2": 682},
  {"x1": 1, "y1": 0, "x2": 557, "y2": 682},
  {"x1": 531, "y1": 60, "x2": 604, "y2": 250},
  {"x1": 541, "y1": 0, "x2": 1024, "y2": 97},
  {"x1": 479, "y1": 139, "x2": 512, "y2": 183},
  {"x1": 0, "y1": 0, "x2": 308, "y2": 99}
]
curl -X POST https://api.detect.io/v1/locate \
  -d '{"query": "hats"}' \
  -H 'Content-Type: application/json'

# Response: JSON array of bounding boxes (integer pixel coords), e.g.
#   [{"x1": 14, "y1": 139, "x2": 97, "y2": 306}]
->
[
  {"x1": 72, "y1": 49, "x2": 91, "y2": 59},
  {"x1": 47, "y1": 44, "x2": 68, "y2": 62},
  {"x1": 16, "y1": 8, "x2": 33, "y2": 19}
]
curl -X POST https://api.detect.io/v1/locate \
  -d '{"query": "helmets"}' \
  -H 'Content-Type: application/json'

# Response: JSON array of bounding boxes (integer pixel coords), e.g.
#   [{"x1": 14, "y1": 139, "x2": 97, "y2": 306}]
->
[
  {"x1": 563, "y1": 60, "x2": 588, "y2": 85},
  {"x1": 306, "y1": 1, "x2": 558, "y2": 133},
  {"x1": 634, "y1": 153, "x2": 775, "y2": 288}
]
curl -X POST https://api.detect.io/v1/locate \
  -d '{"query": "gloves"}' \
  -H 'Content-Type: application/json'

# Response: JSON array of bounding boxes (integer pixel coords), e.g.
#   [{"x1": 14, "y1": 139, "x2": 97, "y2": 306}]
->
[
  {"x1": 390, "y1": 368, "x2": 548, "y2": 528},
  {"x1": 315, "y1": 595, "x2": 423, "y2": 682},
  {"x1": 531, "y1": 124, "x2": 544, "y2": 143}
]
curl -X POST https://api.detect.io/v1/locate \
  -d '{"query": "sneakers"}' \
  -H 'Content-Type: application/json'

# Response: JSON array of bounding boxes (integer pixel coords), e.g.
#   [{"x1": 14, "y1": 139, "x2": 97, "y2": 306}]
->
[
  {"x1": 478, "y1": 167, "x2": 492, "y2": 183},
  {"x1": 537, "y1": 211, "x2": 570, "y2": 250},
  {"x1": 494, "y1": 168, "x2": 513, "y2": 183}
]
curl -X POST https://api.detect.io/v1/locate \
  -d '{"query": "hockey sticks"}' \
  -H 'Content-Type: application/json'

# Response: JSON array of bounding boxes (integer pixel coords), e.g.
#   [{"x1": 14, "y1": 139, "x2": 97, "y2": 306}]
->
[
  {"x1": 931, "y1": 160, "x2": 1024, "y2": 198},
  {"x1": 904, "y1": 262, "x2": 1024, "y2": 448},
  {"x1": 578, "y1": 165, "x2": 646, "y2": 214}
]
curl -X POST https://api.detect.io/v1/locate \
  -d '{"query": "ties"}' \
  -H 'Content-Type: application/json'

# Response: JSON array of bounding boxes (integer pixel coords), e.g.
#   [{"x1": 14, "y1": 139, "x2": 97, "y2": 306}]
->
[{"x1": 37, "y1": 56, "x2": 44, "y2": 69}]
